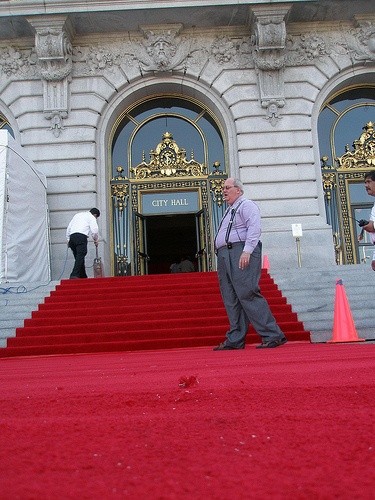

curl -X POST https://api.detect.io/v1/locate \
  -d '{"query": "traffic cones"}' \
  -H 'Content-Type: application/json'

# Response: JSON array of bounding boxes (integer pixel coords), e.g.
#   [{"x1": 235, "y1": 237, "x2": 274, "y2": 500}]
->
[{"x1": 325, "y1": 277, "x2": 366, "y2": 344}]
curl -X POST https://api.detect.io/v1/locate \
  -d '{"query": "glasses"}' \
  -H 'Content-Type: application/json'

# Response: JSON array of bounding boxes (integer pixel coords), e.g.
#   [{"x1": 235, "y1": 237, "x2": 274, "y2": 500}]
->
[{"x1": 221, "y1": 185, "x2": 237, "y2": 191}]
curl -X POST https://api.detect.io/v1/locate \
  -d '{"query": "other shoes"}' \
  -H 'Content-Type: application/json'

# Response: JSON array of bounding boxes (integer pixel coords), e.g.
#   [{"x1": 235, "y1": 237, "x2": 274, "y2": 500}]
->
[
  {"x1": 212, "y1": 345, "x2": 237, "y2": 350},
  {"x1": 255, "y1": 334, "x2": 287, "y2": 348}
]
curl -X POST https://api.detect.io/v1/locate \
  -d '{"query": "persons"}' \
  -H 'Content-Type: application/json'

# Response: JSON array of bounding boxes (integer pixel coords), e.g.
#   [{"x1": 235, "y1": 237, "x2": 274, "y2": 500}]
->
[
  {"x1": 170, "y1": 256, "x2": 198, "y2": 273},
  {"x1": 66, "y1": 208, "x2": 100, "y2": 279},
  {"x1": 212, "y1": 178, "x2": 288, "y2": 350},
  {"x1": 363, "y1": 169, "x2": 375, "y2": 271}
]
